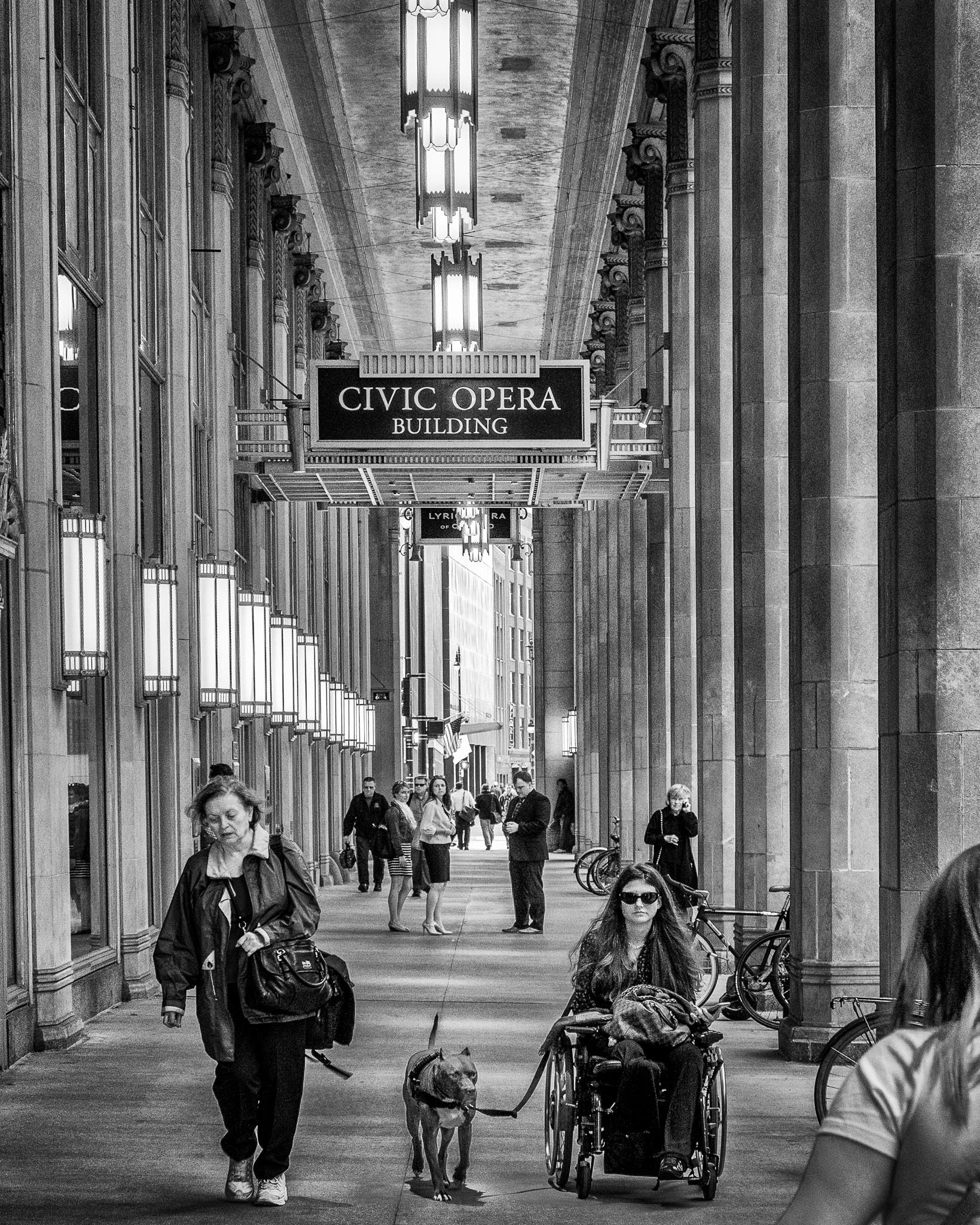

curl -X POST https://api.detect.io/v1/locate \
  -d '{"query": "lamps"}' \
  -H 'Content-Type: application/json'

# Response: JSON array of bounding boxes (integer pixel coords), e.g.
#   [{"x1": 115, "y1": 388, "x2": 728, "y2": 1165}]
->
[
  {"x1": 639, "y1": 403, "x2": 654, "y2": 430},
  {"x1": 265, "y1": 605, "x2": 302, "y2": 738},
  {"x1": 399, "y1": 542, "x2": 422, "y2": 562},
  {"x1": 454, "y1": 502, "x2": 491, "y2": 563},
  {"x1": 399, "y1": 0, "x2": 479, "y2": 245},
  {"x1": 47, "y1": 497, "x2": 113, "y2": 692},
  {"x1": 508, "y1": 543, "x2": 533, "y2": 561},
  {"x1": 235, "y1": 582, "x2": 275, "y2": 727},
  {"x1": 431, "y1": 239, "x2": 483, "y2": 352},
  {"x1": 130, "y1": 551, "x2": 180, "y2": 710},
  {"x1": 289, "y1": 627, "x2": 376, "y2": 758},
  {"x1": 187, "y1": 548, "x2": 239, "y2": 722}
]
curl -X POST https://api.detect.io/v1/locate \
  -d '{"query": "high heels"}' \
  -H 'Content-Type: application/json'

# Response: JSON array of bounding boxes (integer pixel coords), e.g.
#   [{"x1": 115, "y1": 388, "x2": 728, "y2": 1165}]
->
[
  {"x1": 434, "y1": 921, "x2": 453, "y2": 934},
  {"x1": 388, "y1": 920, "x2": 410, "y2": 932},
  {"x1": 422, "y1": 921, "x2": 442, "y2": 935}
]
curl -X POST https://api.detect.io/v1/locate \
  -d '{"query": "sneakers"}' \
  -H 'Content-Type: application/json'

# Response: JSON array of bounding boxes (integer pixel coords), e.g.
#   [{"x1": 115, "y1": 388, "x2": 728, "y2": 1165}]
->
[
  {"x1": 256, "y1": 1174, "x2": 287, "y2": 1206},
  {"x1": 225, "y1": 1156, "x2": 255, "y2": 1203}
]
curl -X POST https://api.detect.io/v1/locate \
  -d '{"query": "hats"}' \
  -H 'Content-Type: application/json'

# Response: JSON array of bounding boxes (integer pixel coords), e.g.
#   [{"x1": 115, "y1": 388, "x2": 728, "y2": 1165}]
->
[{"x1": 481, "y1": 783, "x2": 491, "y2": 792}]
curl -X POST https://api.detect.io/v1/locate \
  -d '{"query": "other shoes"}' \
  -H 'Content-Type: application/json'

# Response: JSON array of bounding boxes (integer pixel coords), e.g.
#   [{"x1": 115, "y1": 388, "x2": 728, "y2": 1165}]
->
[
  {"x1": 374, "y1": 883, "x2": 382, "y2": 891},
  {"x1": 658, "y1": 1157, "x2": 683, "y2": 1178},
  {"x1": 502, "y1": 926, "x2": 519, "y2": 932},
  {"x1": 518, "y1": 927, "x2": 543, "y2": 934},
  {"x1": 358, "y1": 883, "x2": 368, "y2": 892},
  {"x1": 486, "y1": 845, "x2": 491, "y2": 850},
  {"x1": 458, "y1": 845, "x2": 469, "y2": 850}
]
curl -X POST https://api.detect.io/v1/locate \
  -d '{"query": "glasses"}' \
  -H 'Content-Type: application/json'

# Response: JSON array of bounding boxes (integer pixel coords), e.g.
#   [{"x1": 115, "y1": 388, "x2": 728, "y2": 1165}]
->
[
  {"x1": 397, "y1": 781, "x2": 406, "y2": 784},
  {"x1": 415, "y1": 784, "x2": 425, "y2": 787},
  {"x1": 364, "y1": 786, "x2": 373, "y2": 788},
  {"x1": 619, "y1": 891, "x2": 660, "y2": 905}
]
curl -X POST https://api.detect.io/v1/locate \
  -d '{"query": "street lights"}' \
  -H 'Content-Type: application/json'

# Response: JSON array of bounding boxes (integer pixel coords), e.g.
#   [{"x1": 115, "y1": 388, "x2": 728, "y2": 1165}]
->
[{"x1": 453, "y1": 646, "x2": 461, "y2": 717}]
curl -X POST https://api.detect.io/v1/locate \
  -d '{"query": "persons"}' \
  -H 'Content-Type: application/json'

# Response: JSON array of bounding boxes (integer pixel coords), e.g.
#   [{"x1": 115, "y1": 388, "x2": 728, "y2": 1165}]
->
[
  {"x1": 342, "y1": 776, "x2": 518, "y2": 935},
  {"x1": 153, "y1": 763, "x2": 322, "y2": 1206},
  {"x1": 503, "y1": 773, "x2": 552, "y2": 935},
  {"x1": 475, "y1": 784, "x2": 503, "y2": 850},
  {"x1": 644, "y1": 784, "x2": 700, "y2": 910},
  {"x1": 570, "y1": 865, "x2": 703, "y2": 1181},
  {"x1": 779, "y1": 842, "x2": 980, "y2": 1225},
  {"x1": 67, "y1": 782, "x2": 92, "y2": 935},
  {"x1": 552, "y1": 778, "x2": 575, "y2": 854}
]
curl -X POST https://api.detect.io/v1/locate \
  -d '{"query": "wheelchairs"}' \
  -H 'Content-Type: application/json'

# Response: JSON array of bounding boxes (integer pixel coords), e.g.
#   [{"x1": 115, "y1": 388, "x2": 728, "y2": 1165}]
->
[{"x1": 544, "y1": 1002, "x2": 727, "y2": 1200}]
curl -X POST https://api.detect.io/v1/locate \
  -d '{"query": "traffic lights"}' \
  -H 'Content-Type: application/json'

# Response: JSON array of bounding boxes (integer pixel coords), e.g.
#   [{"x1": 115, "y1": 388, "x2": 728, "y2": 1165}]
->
[{"x1": 428, "y1": 720, "x2": 445, "y2": 736}]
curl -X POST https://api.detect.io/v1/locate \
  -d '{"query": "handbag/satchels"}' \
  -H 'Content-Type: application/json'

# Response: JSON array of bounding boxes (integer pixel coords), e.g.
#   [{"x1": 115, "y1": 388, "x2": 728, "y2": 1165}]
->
[
  {"x1": 371, "y1": 825, "x2": 395, "y2": 858},
  {"x1": 459, "y1": 806, "x2": 475, "y2": 824},
  {"x1": 645, "y1": 809, "x2": 663, "y2": 872},
  {"x1": 224, "y1": 880, "x2": 332, "y2": 1013},
  {"x1": 306, "y1": 949, "x2": 354, "y2": 1079},
  {"x1": 340, "y1": 841, "x2": 356, "y2": 869},
  {"x1": 491, "y1": 812, "x2": 502, "y2": 824},
  {"x1": 604, "y1": 983, "x2": 697, "y2": 1047}
]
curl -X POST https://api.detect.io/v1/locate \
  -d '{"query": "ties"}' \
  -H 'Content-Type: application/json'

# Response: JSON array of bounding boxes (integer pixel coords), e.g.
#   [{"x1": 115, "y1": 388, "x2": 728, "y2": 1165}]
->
[{"x1": 511, "y1": 799, "x2": 524, "y2": 820}]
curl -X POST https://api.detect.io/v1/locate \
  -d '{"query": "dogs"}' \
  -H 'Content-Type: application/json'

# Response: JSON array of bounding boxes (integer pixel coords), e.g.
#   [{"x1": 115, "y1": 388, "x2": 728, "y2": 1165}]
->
[{"x1": 402, "y1": 1010, "x2": 478, "y2": 1203}]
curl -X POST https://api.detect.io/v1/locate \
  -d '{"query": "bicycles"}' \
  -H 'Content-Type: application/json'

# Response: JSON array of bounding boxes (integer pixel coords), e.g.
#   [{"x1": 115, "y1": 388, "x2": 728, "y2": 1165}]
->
[
  {"x1": 814, "y1": 998, "x2": 939, "y2": 1121},
  {"x1": 573, "y1": 816, "x2": 621, "y2": 895},
  {"x1": 664, "y1": 874, "x2": 796, "y2": 1030}
]
